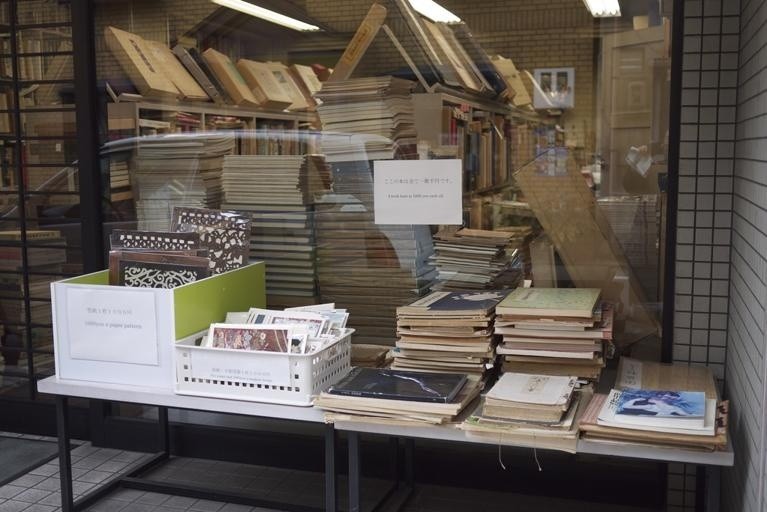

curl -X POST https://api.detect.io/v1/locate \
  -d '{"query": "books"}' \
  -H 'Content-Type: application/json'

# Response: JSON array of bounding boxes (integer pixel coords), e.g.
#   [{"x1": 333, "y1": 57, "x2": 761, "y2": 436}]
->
[
  {"x1": 495, "y1": 289, "x2": 613, "y2": 399},
  {"x1": 578, "y1": 388, "x2": 729, "y2": 453},
  {"x1": 321, "y1": 0, "x2": 560, "y2": 345},
  {"x1": 97, "y1": 9, "x2": 333, "y2": 306},
  {"x1": 315, "y1": 364, "x2": 485, "y2": 427},
  {"x1": 389, "y1": 292, "x2": 508, "y2": 390},
  {"x1": 617, "y1": 356, "x2": 720, "y2": 403},
  {"x1": 455, "y1": 369, "x2": 595, "y2": 454},
  {"x1": 1, "y1": 1, "x2": 83, "y2": 399},
  {"x1": 106, "y1": 207, "x2": 253, "y2": 290}
]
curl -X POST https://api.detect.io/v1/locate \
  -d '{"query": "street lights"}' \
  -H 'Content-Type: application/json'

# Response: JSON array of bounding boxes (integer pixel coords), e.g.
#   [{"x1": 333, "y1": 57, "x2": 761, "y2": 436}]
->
[{"x1": 550, "y1": 86, "x2": 570, "y2": 176}]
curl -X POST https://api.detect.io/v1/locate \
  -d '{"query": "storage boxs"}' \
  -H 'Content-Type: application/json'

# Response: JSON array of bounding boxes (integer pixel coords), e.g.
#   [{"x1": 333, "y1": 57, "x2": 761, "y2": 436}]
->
[
  {"x1": 50, "y1": 262, "x2": 267, "y2": 388},
  {"x1": 174, "y1": 322, "x2": 356, "y2": 407}
]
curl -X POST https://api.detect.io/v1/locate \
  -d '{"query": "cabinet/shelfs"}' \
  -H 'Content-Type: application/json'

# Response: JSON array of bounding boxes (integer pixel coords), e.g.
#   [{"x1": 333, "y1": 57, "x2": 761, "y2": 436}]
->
[
  {"x1": 0, "y1": 23, "x2": 291, "y2": 206},
  {"x1": 38, "y1": 362, "x2": 339, "y2": 512},
  {"x1": 335, "y1": 419, "x2": 735, "y2": 512},
  {"x1": 380, "y1": 20, "x2": 567, "y2": 229},
  {"x1": 107, "y1": 97, "x2": 321, "y2": 155}
]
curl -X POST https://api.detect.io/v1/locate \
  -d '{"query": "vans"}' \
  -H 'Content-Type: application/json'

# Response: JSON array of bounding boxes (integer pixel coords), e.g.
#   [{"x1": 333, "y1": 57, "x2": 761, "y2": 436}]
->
[{"x1": 4, "y1": 131, "x2": 437, "y2": 358}]
